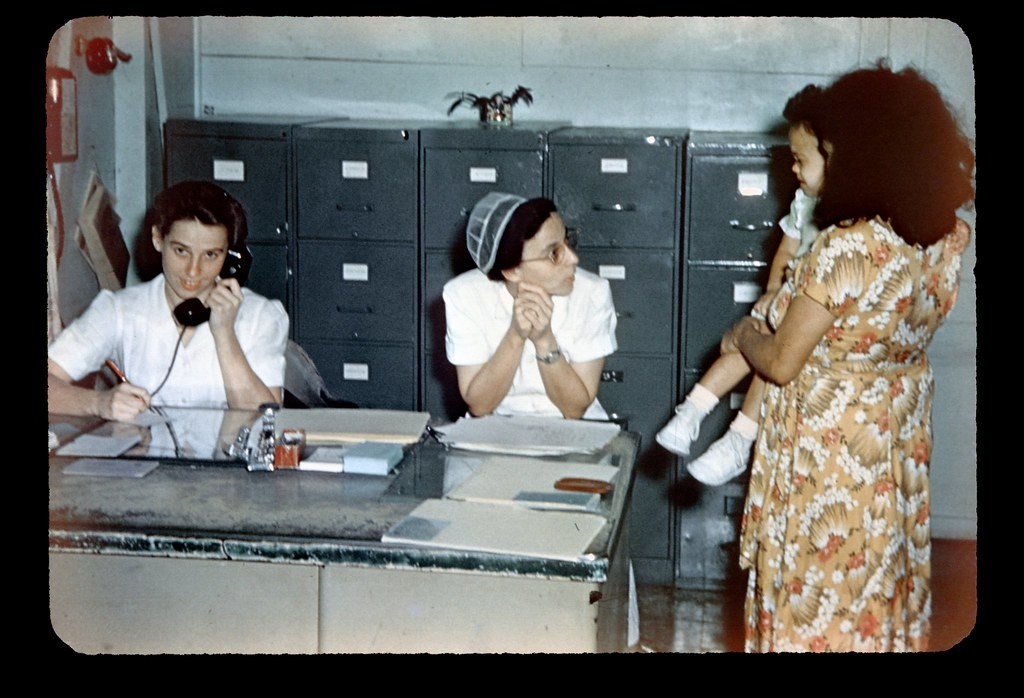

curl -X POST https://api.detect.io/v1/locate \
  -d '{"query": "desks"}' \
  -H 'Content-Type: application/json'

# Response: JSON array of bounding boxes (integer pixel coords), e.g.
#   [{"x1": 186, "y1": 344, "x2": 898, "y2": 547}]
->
[{"x1": 49, "y1": 407, "x2": 641, "y2": 654}]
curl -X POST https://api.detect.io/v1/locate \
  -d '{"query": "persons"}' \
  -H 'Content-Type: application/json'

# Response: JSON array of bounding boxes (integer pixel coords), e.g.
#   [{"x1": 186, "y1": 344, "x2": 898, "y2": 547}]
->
[
  {"x1": 654, "y1": 70, "x2": 975, "y2": 652},
  {"x1": 47, "y1": 181, "x2": 290, "y2": 422},
  {"x1": 442, "y1": 192, "x2": 648, "y2": 652}
]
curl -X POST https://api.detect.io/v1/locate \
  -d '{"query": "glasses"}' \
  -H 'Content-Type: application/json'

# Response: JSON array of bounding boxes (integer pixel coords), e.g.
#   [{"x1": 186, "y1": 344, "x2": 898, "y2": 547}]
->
[{"x1": 521, "y1": 228, "x2": 577, "y2": 265}]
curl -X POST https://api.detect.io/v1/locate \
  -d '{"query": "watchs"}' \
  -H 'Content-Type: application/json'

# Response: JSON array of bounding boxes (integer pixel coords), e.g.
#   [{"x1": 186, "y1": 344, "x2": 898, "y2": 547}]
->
[{"x1": 535, "y1": 350, "x2": 564, "y2": 363}]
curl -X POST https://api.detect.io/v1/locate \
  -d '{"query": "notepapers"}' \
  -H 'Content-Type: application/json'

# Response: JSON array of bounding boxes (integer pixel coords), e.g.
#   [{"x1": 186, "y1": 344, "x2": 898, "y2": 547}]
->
[{"x1": 298, "y1": 440, "x2": 403, "y2": 476}]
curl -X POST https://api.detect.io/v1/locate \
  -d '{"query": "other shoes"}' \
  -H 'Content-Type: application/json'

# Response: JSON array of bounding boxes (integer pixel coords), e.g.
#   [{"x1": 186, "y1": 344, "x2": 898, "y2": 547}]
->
[{"x1": 628, "y1": 645, "x2": 656, "y2": 653}]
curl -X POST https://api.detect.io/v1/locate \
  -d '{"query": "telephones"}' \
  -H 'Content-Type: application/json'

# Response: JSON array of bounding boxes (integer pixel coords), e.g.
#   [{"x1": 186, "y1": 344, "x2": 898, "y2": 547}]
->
[{"x1": 173, "y1": 245, "x2": 250, "y2": 330}]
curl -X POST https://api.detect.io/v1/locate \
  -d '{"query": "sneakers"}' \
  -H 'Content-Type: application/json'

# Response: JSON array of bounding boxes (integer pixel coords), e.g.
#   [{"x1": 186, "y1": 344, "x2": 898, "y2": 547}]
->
[
  {"x1": 687, "y1": 429, "x2": 753, "y2": 487},
  {"x1": 655, "y1": 396, "x2": 710, "y2": 456}
]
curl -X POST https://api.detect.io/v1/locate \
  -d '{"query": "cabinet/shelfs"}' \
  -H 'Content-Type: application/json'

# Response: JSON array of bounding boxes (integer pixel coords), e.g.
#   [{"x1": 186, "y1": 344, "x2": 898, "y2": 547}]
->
[
  {"x1": 292, "y1": 117, "x2": 450, "y2": 408},
  {"x1": 548, "y1": 125, "x2": 689, "y2": 582},
  {"x1": 419, "y1": 119, "x2": 574, "y2": 421},
  {"x1": 163, "y1": 112, "x2": 348, "y2": 409},
  {"x1": 674, "y1": 129, "x2": 801, "y2": 592}
]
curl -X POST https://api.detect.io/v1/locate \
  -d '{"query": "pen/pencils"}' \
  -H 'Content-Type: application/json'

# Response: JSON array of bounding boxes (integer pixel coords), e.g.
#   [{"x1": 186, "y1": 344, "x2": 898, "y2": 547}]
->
[{"x1": 106, "y1": 356, "x2": 154, "y2": 412}]
